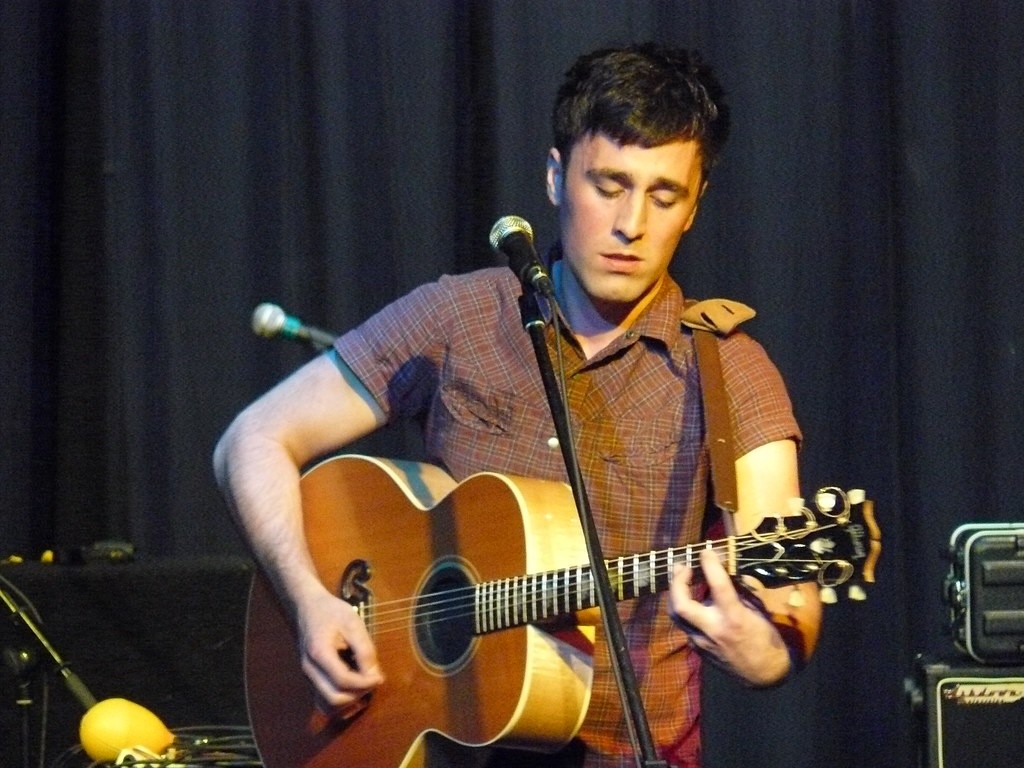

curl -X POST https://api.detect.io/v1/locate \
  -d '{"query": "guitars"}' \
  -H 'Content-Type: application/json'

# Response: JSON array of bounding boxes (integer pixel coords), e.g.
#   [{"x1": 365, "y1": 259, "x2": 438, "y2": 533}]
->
[{"x1": 245, "y1": 454, "x2": 883, "y2": 768}]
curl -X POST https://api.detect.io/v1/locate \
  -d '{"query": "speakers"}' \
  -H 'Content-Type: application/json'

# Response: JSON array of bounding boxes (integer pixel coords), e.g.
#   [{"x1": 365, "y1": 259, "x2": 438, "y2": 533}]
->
[{"x1": 913, "y1": 654, "x2": 1024, "y2": 768}]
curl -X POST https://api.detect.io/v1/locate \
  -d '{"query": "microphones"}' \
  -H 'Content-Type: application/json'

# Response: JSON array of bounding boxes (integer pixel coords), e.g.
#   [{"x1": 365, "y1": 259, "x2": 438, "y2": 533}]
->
[
  {"x1": 489, "y1": 213, "x2": 554, "y2": 295},
  {"x1": 251, "y1": 303, "x2": 338, "y2": 353}
]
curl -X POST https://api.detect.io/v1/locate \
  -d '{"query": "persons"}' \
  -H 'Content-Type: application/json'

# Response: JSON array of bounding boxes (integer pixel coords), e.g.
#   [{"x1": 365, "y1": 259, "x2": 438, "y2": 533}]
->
[{"x1": 212, "y1": 39, "x2": 823, "y2": 768}]
